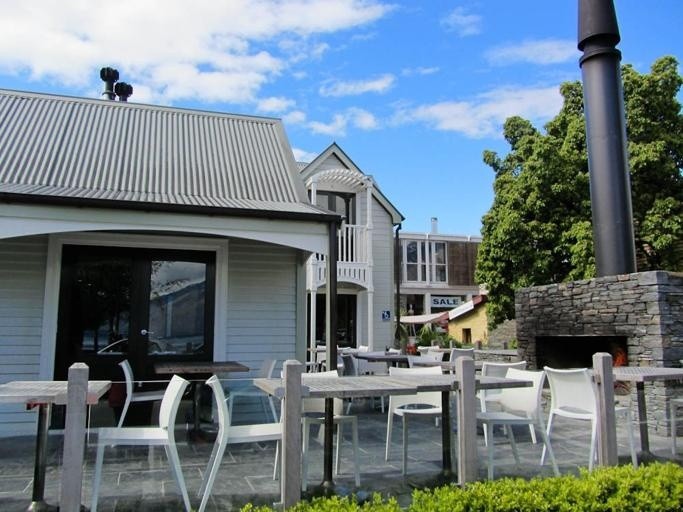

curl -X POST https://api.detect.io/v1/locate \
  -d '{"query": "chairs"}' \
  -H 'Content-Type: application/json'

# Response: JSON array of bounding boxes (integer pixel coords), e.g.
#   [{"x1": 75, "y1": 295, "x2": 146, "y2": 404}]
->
[
  {"x1": 197, "y1": 374, "x2": 282, "y2": 512},
  {"x1": 116, "y1": 360, "x2": 165, "y2": 429},
  {"x1": 229, "y1": 357, "x2": 276, "y2": 424},
  {"x1": 89, "y1": 375, "x2": 192, "y2": 511},
  {"x1": 302, "y1": 341, "x2": 529, "y2": 374},
  {"x1": 271, "y1": 369, "x2": 361, "y2": 488},
  {"x1": 540, "y1": 365, "x2": 639, "y2": 475},
  {"x1": 668, "y1": 399, "x2": 682, "y2": 453},
  {"x1": 476, "y1": 367, "x2": 561, "y2": 481},
  {"x1": 384, "y1": 366, "x2": 454, "y2": 475}
]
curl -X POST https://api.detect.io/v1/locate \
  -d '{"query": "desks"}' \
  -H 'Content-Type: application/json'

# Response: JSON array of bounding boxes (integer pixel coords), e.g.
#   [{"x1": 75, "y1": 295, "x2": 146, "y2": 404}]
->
[
  {"x1": 156, "y1": 359, "x2": 248, "y2": 455},
  {"x1": 586, "y1": 365, "x2": 683, "y2": 463},
  {"x1": 378, "y1": 371, "x2": 534, "y2": 490},
  {"x1": 252, "y1": 375, "x2": 417, "y2": 503},
  {"x1": 1, "y1": 380, "x2": 113, "y2": 511}
]
